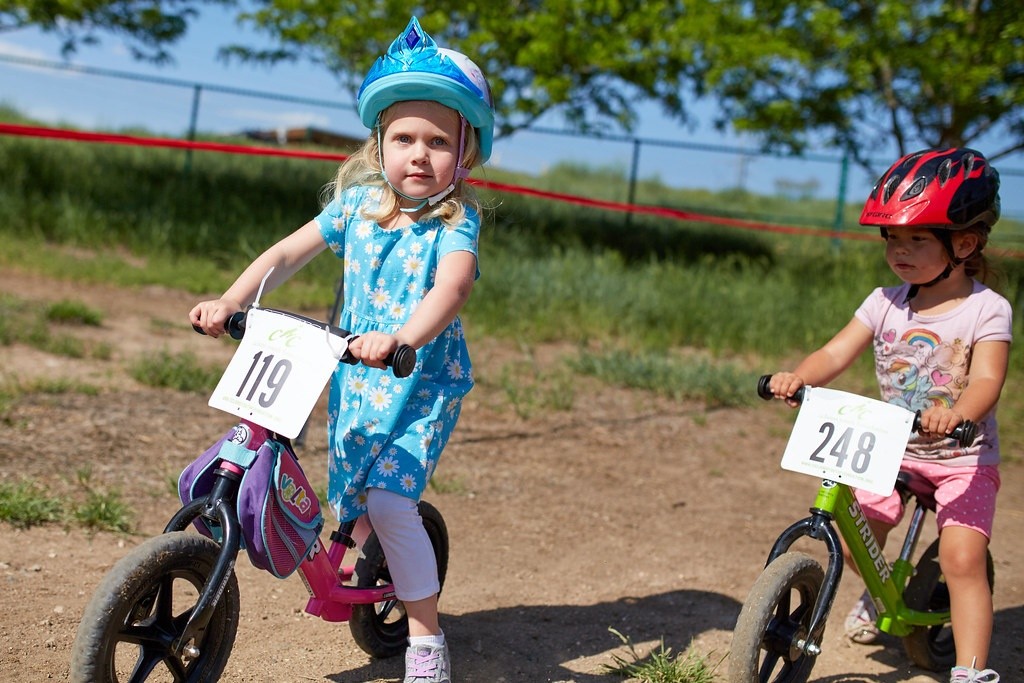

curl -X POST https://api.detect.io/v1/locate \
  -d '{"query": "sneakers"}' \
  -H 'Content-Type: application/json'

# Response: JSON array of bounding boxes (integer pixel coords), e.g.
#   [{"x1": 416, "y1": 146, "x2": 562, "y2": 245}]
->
[
  {"x1": 845, "y1": 589, "x2": 886, "y2": 643},
  {"x1": 403, "y1": 640, "x2": 451, "y2": 683},
  {"x1": 949, "y1": 656, "x2": 1000, "y2": 683}
]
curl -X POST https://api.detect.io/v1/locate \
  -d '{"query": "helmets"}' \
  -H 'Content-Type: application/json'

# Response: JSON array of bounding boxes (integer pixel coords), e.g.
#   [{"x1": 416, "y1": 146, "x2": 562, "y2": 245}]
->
[
  {"x1": 357, "y1": 16, "x2": 495, "y2": 167},
  {"x1": 859, "y1": 146, "x2": 1001, "y2": 230}
]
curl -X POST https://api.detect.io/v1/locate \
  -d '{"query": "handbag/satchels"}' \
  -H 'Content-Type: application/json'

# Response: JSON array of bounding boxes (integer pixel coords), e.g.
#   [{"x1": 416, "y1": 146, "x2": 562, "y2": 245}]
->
[{"x1": 178, "y1": 425, "x2": 325, "y2": 579}]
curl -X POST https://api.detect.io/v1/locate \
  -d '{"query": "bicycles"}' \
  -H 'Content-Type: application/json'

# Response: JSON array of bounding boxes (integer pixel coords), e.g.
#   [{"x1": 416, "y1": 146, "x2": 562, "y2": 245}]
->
[
  {"x1": 69, "y1": 301, "x2": 449, "y2": 683},
  {"x1": 724, "y1": 375, "x2": 996, "y2": 683}
]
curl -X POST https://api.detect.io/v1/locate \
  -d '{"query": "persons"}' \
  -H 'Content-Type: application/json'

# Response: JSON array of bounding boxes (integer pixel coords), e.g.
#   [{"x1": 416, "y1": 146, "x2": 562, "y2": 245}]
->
[
  {"x1": 188, "y1": 15, "x2": 493, "y2": 683},
  {"x1": 769, "y1": 148, "x2": 1014, "y2": 683}
]
